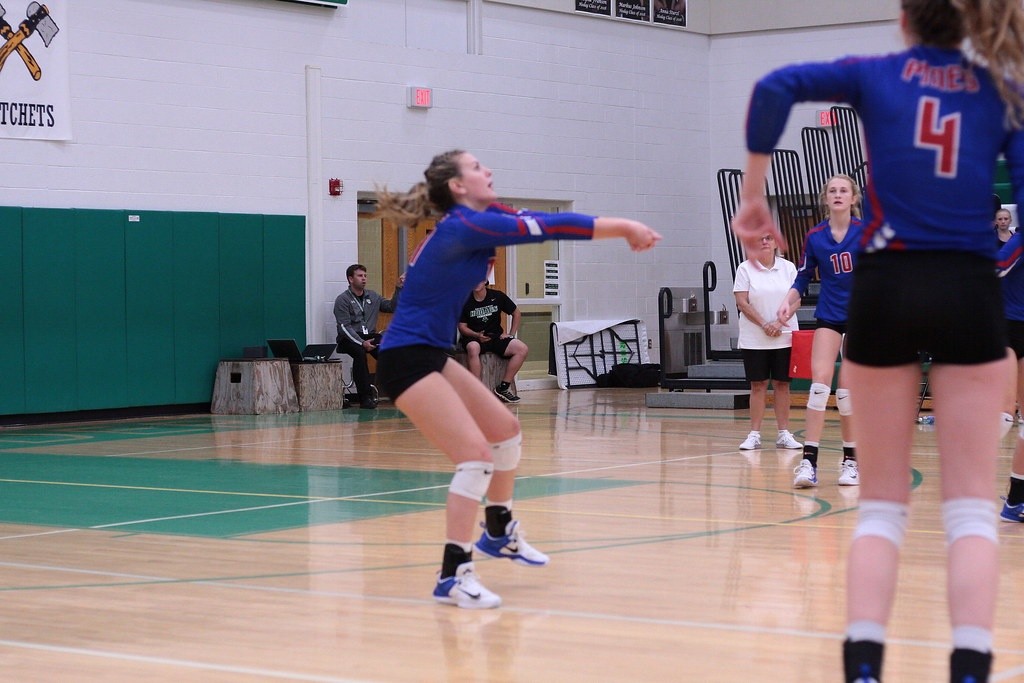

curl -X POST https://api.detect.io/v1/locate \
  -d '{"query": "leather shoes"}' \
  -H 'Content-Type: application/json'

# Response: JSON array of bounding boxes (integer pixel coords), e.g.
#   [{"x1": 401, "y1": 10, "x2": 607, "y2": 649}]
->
[{"x1": 360, "y1": 397, "x2": 377, "y2": 408}]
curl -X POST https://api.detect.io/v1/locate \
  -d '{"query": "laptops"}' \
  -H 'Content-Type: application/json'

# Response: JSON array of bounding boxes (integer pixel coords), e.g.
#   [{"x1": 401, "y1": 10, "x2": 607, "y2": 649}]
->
[
  {"x1": 302, "y1": 343, "x2": 337, "y2": 360},
  {"x1": 267, "y1": 338, "x2": 342, "y2": 363}
]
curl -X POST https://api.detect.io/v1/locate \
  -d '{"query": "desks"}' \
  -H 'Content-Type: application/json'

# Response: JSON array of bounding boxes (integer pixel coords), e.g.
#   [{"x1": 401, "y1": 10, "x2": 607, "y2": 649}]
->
[{"x1": 553, "y1": 321, "x2": 652, "y2": 390}]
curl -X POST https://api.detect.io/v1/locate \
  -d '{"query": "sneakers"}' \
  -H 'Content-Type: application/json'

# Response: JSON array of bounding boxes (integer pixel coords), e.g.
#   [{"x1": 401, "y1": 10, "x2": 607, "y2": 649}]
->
[
  {"x1": 776, "y1": 432, "x2": 804, "y2": 448},
  {"x1": 474, "y1": 521, "x2": 547, "y2": 566},
  {"x1": 494, "y1": 386, "x2": 520, "y2": 402},
  {"x1": 999, "y1": 500, "x2": 1024, "y2": 521},
  {"x1": 839, "y1": 485, "x2": 860, "y2": 499},
  {"x1": 433, "y1": 561, "x2": 501, "y2": 609},
  {"x1": 794, "y1": 459, "x2": 818, "y2": 485},
  {"x1": 776, "y1": 450, "x2": 802, "y2": 463},
  {"x1": 838, "y1": 460, "x2": 860, "y2": 485},
  {"x1": 739, "y1": 449, "x2": 761, "y2": 462},
  {"x1": 794, "y1": 489, "x2": 814, "y2": 509},
  {"x1": 738, "y1": 433, "x2": 760, "y2": 448}
]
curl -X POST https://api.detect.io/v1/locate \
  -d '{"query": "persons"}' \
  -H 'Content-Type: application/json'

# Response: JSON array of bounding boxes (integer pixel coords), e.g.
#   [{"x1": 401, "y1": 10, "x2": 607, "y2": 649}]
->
[
  {"x1": 333, "y1": 264, "x2": 407, "y2": 409},
  {"x1": 991, "y1": 209, "x2": 1014, "y2": 250},
  {"x1": 733, "y1": 231, "x2": 803, "y2": 449},
  {"x1": 777, "y1": 173, "x2": 862, "y2": 486},
  {"x1": 458, "y1": 276, "x2": 528, "y2": 403},
  {"x1": 995, "y1": 233, "x2": 1024, "y2": 522},
  {"x1": 371, "y1": 150, "x2": 662, "y2": 611},
  {"x1": 732, "y1": 0, "x2": 1024, "y2": 682}
]
